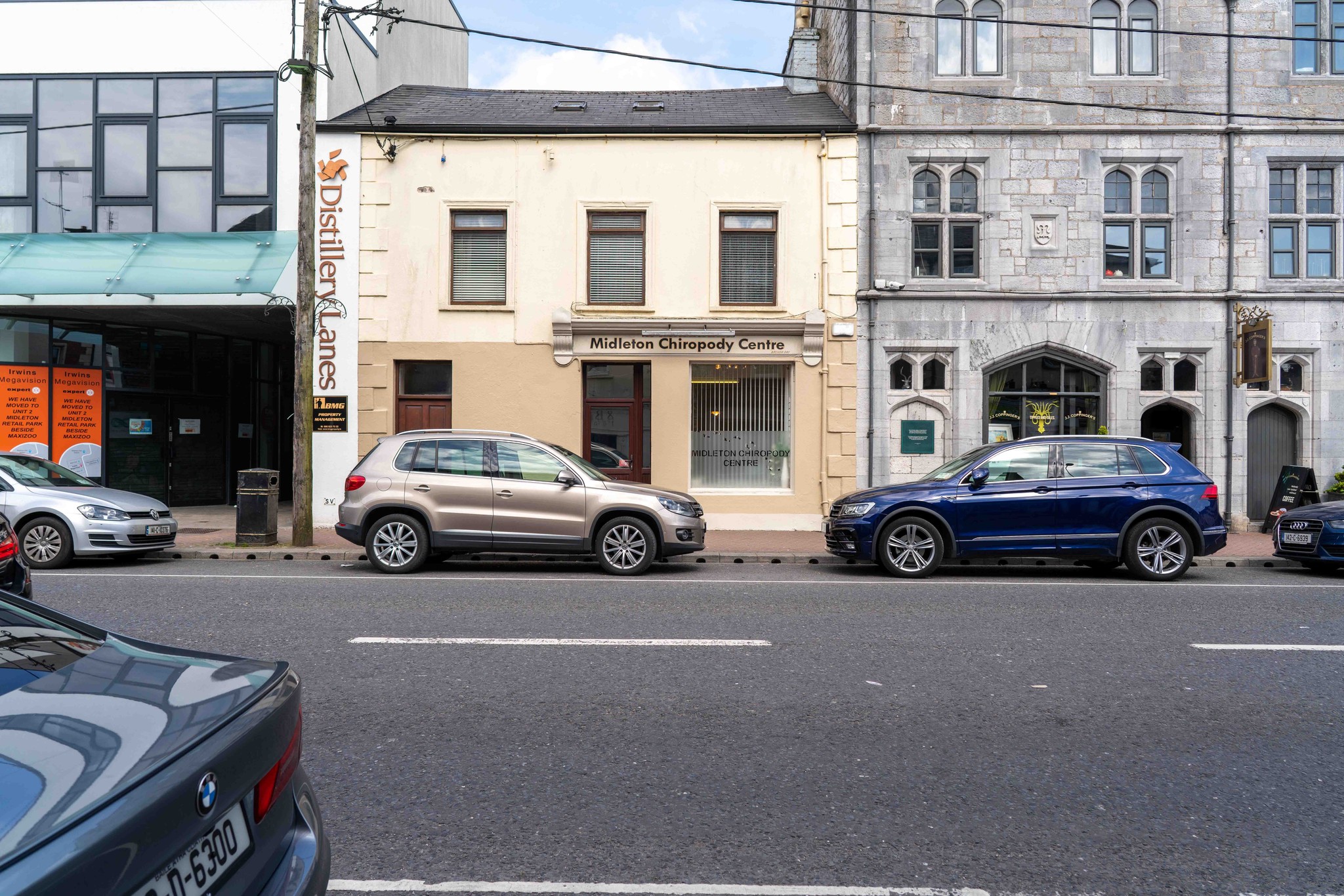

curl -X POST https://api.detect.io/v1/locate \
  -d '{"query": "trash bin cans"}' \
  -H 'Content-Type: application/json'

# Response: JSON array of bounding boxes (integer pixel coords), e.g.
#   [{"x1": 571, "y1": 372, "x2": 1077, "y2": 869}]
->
[{"x1": 235, "y1": 468, "x2": 280, "y2": 547}]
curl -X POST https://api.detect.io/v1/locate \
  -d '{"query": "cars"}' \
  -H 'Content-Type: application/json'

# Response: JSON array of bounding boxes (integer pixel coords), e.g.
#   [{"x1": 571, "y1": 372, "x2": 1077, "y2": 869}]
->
[
  {"x1": 1271, "y1": 500, "x2": 1344, "y2": 575},
  {"x1": 0, "y1": 511, "x2": 35, "y2": 603},
  {"x1": 0, "y1": 586, "x2": 336, "y2": 896},
  {"x1": 0, "y1": 450, "x2": 181, "y2": 570}
]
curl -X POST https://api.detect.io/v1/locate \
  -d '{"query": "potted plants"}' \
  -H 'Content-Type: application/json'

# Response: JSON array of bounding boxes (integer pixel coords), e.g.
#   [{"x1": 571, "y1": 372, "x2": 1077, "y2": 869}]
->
[{"x1": 1323, "y1": 464, "x2": 1344, "y2": 502}]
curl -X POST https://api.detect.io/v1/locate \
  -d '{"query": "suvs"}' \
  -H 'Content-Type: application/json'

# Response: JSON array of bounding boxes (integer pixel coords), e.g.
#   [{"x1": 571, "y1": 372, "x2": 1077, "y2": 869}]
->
[
  {"x1": 333, "y1": 428, "x2": 708, "y2": 577},
  {"x1": 590, "y1": 441, "x2": 630, "y2": 469},
  {"x1": 822, "y1": 433, "x2": 1230, "y2": 584}
]
